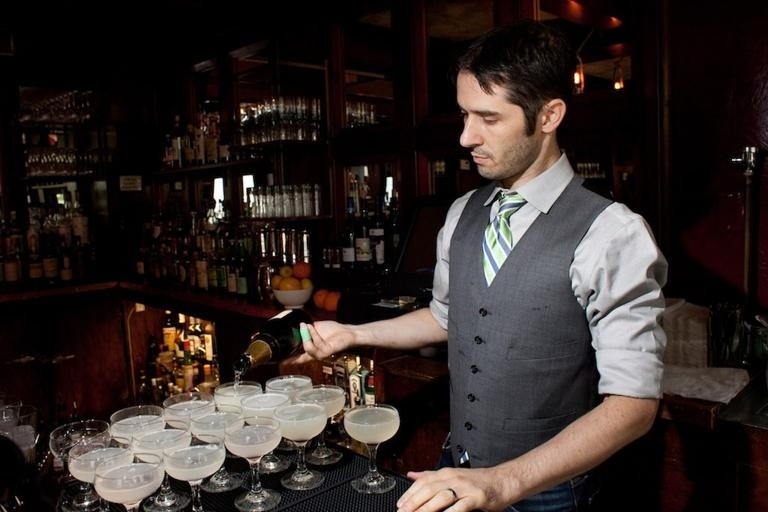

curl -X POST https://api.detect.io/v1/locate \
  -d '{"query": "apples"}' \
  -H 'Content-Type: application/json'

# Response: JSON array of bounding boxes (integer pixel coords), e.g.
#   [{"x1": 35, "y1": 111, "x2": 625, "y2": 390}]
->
[
  {"x1": 280, "y1": 266, "x2": 293, "y2": 278},
  {"x1": 293, "y1": 261, "x2": 310, "y2": 279}
]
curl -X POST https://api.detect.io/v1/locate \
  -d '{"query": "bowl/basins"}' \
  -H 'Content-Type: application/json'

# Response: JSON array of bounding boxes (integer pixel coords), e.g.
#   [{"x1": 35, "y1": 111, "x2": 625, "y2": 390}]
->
[{"x1": 271, "y1": 287, "x2": 313, "y2": 310}]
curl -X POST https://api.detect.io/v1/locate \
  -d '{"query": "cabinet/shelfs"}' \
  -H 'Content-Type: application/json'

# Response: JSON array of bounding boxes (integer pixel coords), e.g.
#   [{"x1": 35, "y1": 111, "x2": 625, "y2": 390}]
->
[{"x1": 1, "y1": 1, "x2": 425, "y2": 316}]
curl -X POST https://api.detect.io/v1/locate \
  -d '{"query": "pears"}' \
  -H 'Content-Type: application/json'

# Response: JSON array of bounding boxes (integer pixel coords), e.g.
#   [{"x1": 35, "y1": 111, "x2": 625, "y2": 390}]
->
[{"x1": 271, "y1": 276, "x2": 312, "y2": 290}]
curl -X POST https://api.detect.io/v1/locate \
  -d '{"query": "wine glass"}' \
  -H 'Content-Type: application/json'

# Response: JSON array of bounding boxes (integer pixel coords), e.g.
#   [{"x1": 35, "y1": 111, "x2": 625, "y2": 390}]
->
[{"x1": 48, "y1": 376, "x2": 400, "y2": 511}]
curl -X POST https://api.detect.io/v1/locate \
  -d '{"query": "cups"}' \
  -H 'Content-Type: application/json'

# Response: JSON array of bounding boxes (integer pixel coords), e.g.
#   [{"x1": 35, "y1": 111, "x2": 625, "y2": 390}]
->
[
  {"x1": 24, "y1": 151, "x2": 94, "y2": 177},
  {"x1": 0, "y1": 397, "x2": 36, "y2": 465}
]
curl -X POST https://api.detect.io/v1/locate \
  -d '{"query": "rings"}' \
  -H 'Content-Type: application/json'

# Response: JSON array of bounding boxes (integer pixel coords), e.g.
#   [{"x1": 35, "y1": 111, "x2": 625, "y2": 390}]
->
[{"x1": 446, "y1": 485, "x2": 460, "y2": 501}]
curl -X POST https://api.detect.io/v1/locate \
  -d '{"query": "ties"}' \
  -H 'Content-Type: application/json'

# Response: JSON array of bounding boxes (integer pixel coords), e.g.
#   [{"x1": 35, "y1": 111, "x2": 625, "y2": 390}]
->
[{"x1": 480, "y1": 192, "x2": 528, "y2": 288}]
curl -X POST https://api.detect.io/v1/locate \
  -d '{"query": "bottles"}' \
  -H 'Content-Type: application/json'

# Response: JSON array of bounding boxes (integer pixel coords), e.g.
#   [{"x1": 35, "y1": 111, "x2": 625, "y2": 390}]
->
[
  {"x1": 0, "y1": 190, "x2": 88, "y2": 285},
  {"x1": 136, "y1": 95, "x2": 391, "y2": 304},
  {"x1": 139, "y1": 311, "x2": 220, "y2": 390},
  {"x1": 233, "y1": 310, "x2": 316, "y2": 371}
]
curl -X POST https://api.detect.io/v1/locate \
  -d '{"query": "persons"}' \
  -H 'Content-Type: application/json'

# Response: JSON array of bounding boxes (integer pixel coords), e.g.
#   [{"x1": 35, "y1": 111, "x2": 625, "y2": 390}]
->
[{"x1": 274, "y1": 20, "x2": 672, "y2": 511}]
